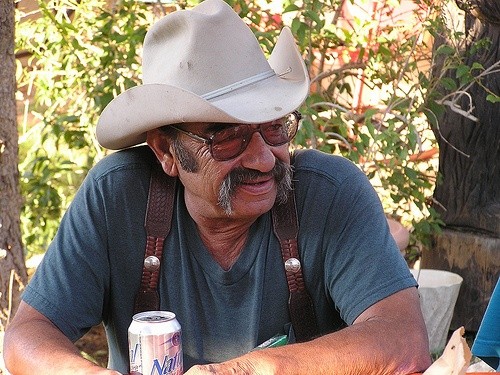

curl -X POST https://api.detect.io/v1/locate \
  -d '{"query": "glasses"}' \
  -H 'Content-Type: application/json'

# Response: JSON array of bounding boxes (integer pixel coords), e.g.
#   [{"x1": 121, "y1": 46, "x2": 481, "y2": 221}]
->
[{"x1": 163, "y1": 108, "x2": 302, "y2": 162}]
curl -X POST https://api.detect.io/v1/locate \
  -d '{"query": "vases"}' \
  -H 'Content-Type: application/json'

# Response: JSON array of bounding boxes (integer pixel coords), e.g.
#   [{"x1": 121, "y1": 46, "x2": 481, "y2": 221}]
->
[{"x1": 409, "y1": 268, "x2": 463, "y2": 357}]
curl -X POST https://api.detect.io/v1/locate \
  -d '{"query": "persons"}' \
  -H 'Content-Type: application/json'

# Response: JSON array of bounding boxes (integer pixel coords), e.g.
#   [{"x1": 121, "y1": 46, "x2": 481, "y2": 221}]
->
[{"x1": 1, "y1": 0, "x2": 434, "y2": 375}]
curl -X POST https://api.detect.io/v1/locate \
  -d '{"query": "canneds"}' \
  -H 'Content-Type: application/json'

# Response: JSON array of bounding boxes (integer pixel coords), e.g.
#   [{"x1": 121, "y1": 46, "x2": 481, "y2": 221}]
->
[{"x1": 127, "y1": 311, "x2": 184, "y2": 375}]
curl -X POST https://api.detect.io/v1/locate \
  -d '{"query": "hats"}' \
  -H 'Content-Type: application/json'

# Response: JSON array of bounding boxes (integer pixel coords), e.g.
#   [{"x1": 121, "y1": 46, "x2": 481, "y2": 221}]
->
[{"x1": 96, "y1": 0, "x2": 310, "y2": 150}]
